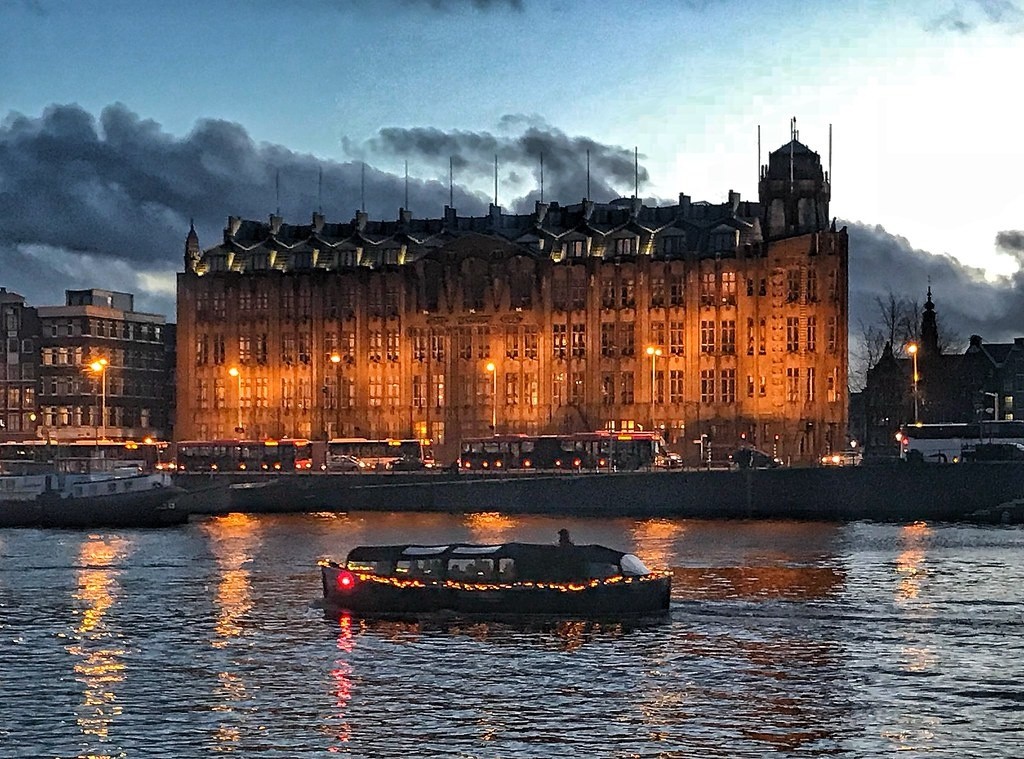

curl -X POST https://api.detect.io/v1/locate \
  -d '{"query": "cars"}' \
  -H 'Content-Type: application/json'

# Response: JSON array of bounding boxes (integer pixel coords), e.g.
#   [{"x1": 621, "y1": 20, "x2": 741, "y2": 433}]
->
[
  {"x1": 387, "y1": 454, "x2": 426, "y2": 471},
  {"x1": 727, "y1": 446, "x2": 784, "y2": 469},
  {"x1": 820, "y1": 449, "x2": 864, "y2": 468},
  {"x1": 328, "y1": 455, "x2": 365, "y2": 472}
]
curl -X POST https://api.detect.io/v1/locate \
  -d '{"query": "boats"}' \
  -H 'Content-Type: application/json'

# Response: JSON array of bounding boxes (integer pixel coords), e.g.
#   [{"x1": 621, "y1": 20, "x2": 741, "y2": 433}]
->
[
  {"x1": 306, "y1": 527, "x2": 675, "y2": 621},
  {"x1": 0, "y1": 463, "x2": 190, "y2": 528}
]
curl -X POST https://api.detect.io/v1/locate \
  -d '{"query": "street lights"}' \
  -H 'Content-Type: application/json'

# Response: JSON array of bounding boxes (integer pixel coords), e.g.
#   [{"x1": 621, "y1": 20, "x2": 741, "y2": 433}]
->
[
  {"x1": 908, "y1": 344, "x2": 919, "y2": 424},
  {"x1": 90, "y1": 358, "x2": 109, "y2": 441},
  {"x1": 486, "y1": 363, "x2": 498, "y2": 435},
  {"x1": 646, "y1": 347, "x2": 663, "y2": 431},
  {"x1": 229, "y1": 367, "x2": 244, "y2": 440}
]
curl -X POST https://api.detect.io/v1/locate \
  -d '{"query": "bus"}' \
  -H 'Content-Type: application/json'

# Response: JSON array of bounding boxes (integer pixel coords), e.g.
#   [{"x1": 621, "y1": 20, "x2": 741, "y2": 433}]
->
[
  {"x1": 176, "y1": 437, "x2": 314, "y2": 472},
  {"x1": 459, "y1": 431, "x2": 667, "y2": 470},
  {"x1": 0, "y1": 437, "x2": 178, "y2": 472},
  {"x1": 896, "y1": 418, "x2": 1024, "y2": 465},
  {"x1": 322, "y1": 437, "x2": 436, "y2": 471}
]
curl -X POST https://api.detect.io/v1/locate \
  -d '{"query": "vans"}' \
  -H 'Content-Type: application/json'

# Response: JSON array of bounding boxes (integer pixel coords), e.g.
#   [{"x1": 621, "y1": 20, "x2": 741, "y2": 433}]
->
[{"x1": 974, "y1": 442, "x2": 1024, "y2": 465}]
